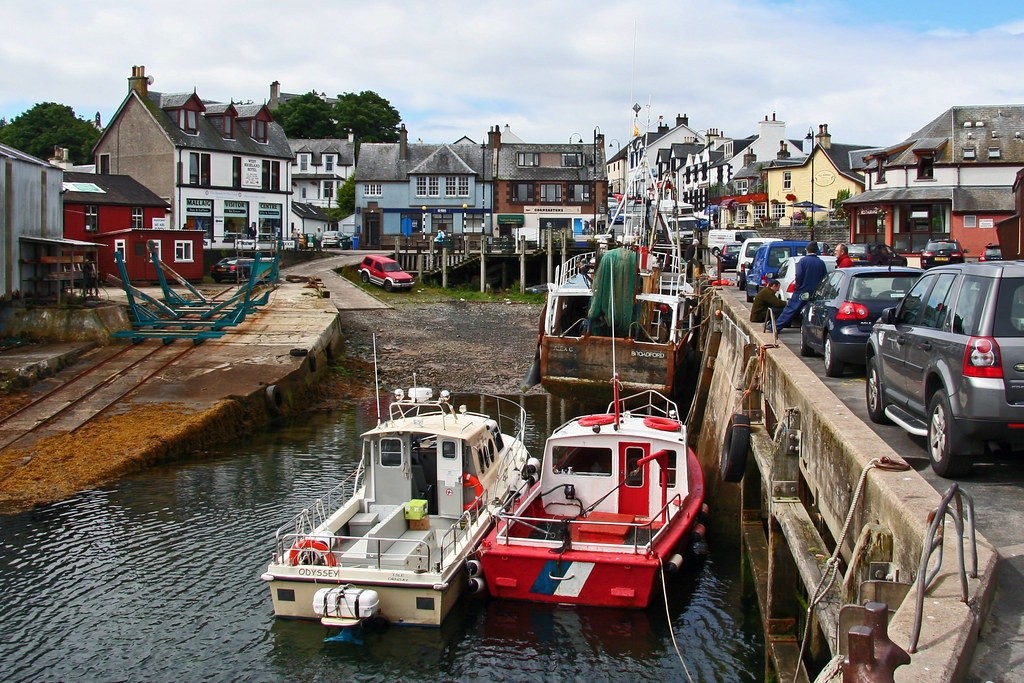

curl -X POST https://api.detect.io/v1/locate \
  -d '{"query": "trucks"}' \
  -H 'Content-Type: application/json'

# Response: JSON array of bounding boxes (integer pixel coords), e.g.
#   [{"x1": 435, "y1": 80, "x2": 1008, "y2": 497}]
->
[{"x1": 627, "y1": 200, "x2": 697, "y2": 243}]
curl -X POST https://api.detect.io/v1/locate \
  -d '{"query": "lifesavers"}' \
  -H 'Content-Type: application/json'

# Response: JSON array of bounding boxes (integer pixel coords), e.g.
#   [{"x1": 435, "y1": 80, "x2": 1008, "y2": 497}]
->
[
  {"x1": 290, "y1": 540, "x2": 337, "y2": 567},
  {"x1": 643, "y1": 417, "x2": 680, "y2": 432},
  {"x1": 720, "y1": 414, "x2": 751, "y2": 484},
  {"x1": 578, "y1": 415, "x2": 615, "y2": 426},
  {"x1": 462, "y1": 472, "x2": 483, "y2": 513}
]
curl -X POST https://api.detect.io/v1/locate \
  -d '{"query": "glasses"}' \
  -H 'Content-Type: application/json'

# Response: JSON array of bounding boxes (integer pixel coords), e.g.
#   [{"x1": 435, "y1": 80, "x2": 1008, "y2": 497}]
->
[{"x1": 833, "y1": 250, "x2": 842, "y2": 252}]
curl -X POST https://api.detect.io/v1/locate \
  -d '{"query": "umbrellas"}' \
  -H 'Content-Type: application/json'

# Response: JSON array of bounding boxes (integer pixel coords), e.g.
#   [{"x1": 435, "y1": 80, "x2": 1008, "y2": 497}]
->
[{"x1": 786, "y1": 201, "x2": 828, "y2": 220}]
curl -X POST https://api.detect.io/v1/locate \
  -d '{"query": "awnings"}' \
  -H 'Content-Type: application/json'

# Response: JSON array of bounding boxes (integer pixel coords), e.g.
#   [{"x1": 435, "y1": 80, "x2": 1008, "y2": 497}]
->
[
  {"x1": 497, "y1": 214, "x2": 524, "y2": 225},
  {"x1": 721, "y1": 199, "x2": 735, "y2": 211},
  {"x1": 704, "y1": 205, "x2": 718, "y2": 215}
]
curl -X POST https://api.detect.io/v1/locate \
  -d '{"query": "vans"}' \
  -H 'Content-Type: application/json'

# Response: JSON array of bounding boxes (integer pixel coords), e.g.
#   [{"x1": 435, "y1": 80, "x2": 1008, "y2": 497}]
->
[
  {"x1": 708, "y1": 229, "x2": 763, "y2": 258},
  {"x1": 735, "y1": 237, "x2": 821, "y2": 302}
]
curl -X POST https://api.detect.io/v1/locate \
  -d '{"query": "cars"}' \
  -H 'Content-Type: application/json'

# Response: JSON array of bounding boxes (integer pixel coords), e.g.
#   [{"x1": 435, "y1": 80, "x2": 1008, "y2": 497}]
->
[
  {"x1": 608, "y1": 197, "x2": 624, "y2": 224},
  {"x1": 357, "y1": 255, "x2": 415, "y2": 292},
  {"x1": 817, "y1": 242, "x2": 908, "y2": 266},
  {"x1": 210, "y1": 257, "x2": 251, "y2": 282},
  {"x1": 719, "y1": 244, "x2": 743, "y2": 273},
  {"x1": 765, "y1": 255, "x2": 837, "y2": 305},
  {"x1": 322, "y1": 231, "x2": 351, "y2": 247},
  {"x1": 798, "y1": 265, "x2": 926, "y2": 377},
  {"x1": 864, "y1": 260, "x2": 1024, "y2": 478}
]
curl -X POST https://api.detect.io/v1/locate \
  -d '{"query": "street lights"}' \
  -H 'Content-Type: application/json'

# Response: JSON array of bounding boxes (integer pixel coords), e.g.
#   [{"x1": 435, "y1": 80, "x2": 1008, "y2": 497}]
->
[
  {"x1": 593, "y1": 126, "x2": 602, "y2": 234},
  {"x1": 693, "y1": 130, "x2": 711, "y2": 231},
  {"x1": 804, "y1": 127, "x2": 814, "y2": 241},
  {"x1": 481, "y1": 139, "x2": 486, "y2": 237}
]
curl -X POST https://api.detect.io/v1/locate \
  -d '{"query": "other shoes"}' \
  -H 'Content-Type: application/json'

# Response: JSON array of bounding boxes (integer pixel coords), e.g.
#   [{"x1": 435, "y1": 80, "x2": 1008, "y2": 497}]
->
[{"x1": 762, "y1": 323, "x2": 770, "y2": 330}]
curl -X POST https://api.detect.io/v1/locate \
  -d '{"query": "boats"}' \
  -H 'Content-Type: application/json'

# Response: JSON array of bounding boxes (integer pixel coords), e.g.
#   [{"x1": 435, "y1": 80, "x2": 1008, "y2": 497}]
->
[
  {"x1": 539, "y1": 94, "x2": 700, "y2": 403},
  {"x1": 261, "y1": 388, "x2": 539, "y2": 646},
  {"x1": 475, "y1": 390, "x2": 704, "y2": 609}
]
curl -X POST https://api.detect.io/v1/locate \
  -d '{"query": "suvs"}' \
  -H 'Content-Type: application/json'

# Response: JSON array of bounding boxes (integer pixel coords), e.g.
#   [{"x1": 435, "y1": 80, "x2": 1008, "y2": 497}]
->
[{"x1": 921, "y1": 238, "x2": 1002, "y2": 270}]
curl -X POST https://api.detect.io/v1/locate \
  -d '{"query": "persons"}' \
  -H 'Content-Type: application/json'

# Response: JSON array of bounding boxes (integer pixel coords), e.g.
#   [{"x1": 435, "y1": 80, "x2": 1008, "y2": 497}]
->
[
  {"x1": 183, "y1": 224, "x2": 189, "y2": 230},
  {"x1": 834, "y1": 242, "x2": 854, "y2": 269},
  {"x1": 249, "y1": 223, "x2": 257, "y2": 249},
  {"x1": 436, "y1": 230, "x2": 443, "y2": 253},
  {"x1": 686, "y1": 239, "x2": 702, "y2": 277},
  {"x1": 762, "y1": 240, "x2": 828, "y2": 335},
  {"x1": 750, "y1": 279, "x2": 788, "y2": 324},
  {"x1": 315, "y1": 228, "x2": 323, "y2": 252},
  {"x1": 292, "y1": 227, "x2": 301, "y2": 237}
]
